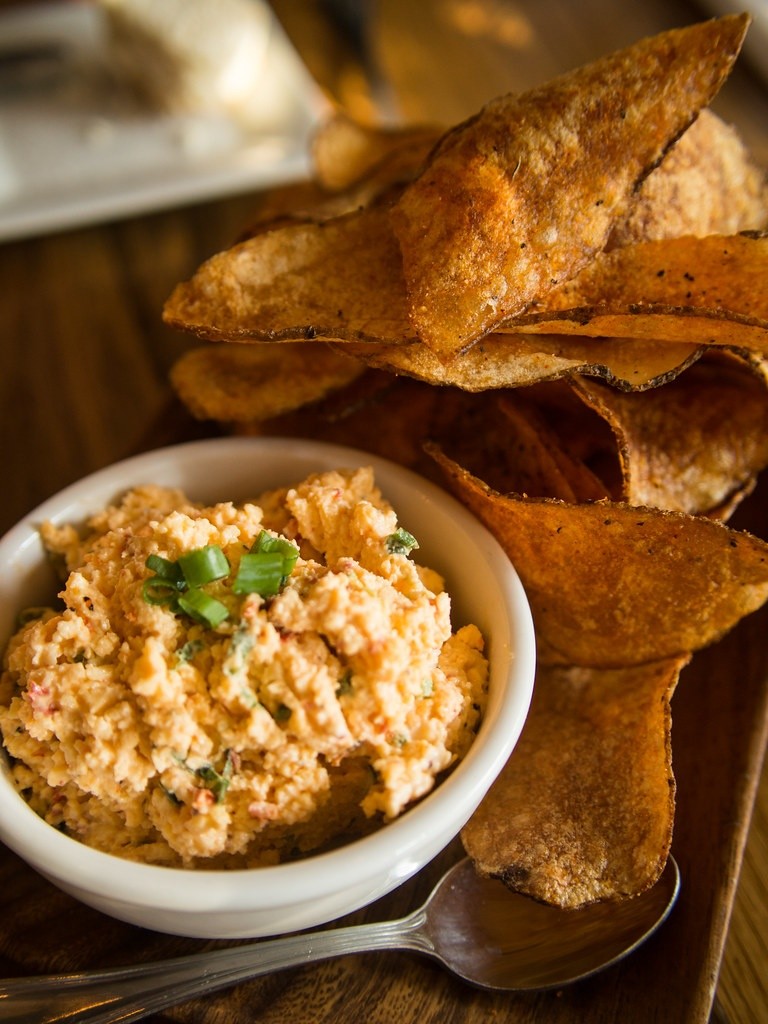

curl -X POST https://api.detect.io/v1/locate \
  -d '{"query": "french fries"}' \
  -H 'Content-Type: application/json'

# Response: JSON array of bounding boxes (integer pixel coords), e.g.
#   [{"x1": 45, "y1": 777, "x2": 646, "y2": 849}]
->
[{"x1": 157, "y1": 13, "x2": 766, "y2": 910}]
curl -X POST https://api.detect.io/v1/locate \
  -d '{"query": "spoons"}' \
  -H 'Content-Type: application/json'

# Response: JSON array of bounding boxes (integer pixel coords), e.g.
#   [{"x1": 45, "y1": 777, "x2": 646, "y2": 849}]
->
[{"x1": 1, "y1": 846, "x2": 682, "y2": 1024}]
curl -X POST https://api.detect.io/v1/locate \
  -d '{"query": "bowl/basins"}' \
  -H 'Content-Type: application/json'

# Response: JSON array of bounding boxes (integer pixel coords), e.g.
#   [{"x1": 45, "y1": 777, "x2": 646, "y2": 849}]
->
[{"x1": 0, "y1": 438, "x2": 543, "y2": 946}]
